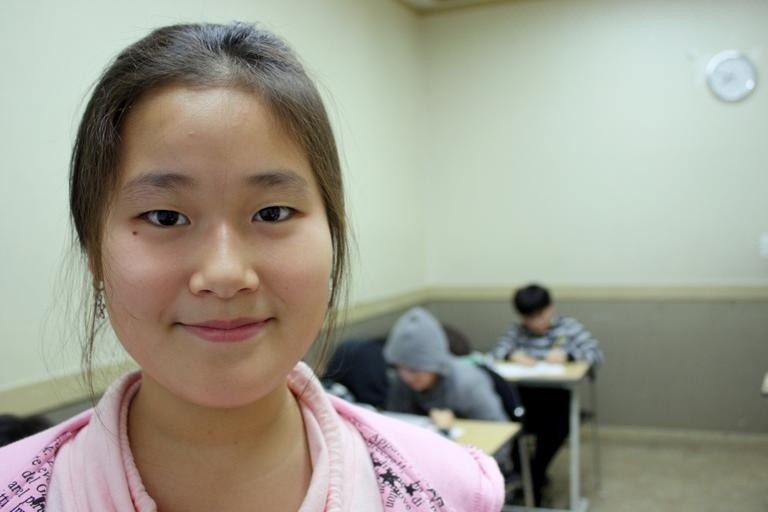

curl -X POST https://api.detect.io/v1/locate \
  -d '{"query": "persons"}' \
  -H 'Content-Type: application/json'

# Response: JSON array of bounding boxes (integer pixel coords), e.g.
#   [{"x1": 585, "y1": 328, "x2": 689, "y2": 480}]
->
[
  {"x1": 488, "y1": 279, "x2": 604, "y2": 494},
  {"x1": 383, "y1": 308, "x2": 514, "y2": 476},
  {"x1": 1, "y1": 21, "x2": 507, "y2": 512}
]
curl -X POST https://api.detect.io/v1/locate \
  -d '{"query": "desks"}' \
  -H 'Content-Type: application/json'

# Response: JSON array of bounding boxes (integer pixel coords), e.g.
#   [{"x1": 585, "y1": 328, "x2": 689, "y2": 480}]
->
[
  {"x1": 376, "y1": 410, "x2": 535, "y2": 511},
  {"x1": 469, "y1": 355, "x2": 608, "y2": 511}
]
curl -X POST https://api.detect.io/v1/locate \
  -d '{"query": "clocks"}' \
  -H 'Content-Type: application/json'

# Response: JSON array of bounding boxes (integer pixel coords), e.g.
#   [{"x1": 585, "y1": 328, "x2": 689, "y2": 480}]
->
[{"x1": 704, "y1": 50, "x2": 760, "y2": 105}]
